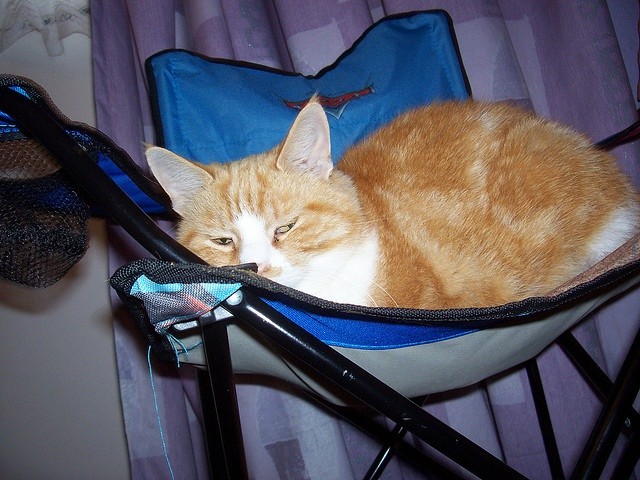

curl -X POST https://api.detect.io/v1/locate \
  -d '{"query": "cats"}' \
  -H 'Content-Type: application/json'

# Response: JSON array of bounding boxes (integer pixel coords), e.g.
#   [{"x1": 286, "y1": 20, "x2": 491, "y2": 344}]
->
[{"x1": 140, "y1": 90, "x2": 640, "y2": 311}]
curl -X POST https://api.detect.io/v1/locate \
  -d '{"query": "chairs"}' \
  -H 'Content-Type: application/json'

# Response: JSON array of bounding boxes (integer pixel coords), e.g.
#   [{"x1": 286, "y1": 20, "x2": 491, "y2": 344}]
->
[{"x1": 1, "y1": 0, "x2": 639, "y2": 479}]
